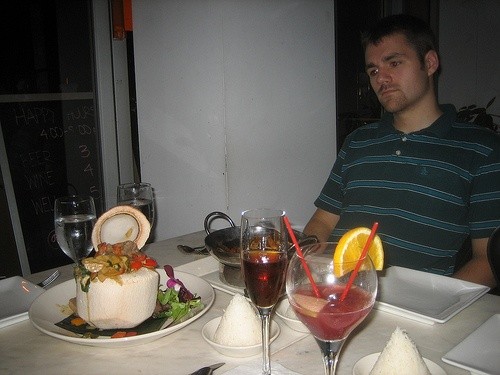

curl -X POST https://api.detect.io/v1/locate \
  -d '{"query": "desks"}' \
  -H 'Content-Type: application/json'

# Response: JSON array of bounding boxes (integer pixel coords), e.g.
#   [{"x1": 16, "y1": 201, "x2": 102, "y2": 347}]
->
[{"x1": 0, "y1": 229, "x2": 499, "y2": 375}]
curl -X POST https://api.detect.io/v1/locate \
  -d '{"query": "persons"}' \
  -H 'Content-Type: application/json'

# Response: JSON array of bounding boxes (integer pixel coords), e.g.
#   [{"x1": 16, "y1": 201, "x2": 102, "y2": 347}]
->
[{"x1": 302, "y1": 13, "x2": 500, "y2": 289}]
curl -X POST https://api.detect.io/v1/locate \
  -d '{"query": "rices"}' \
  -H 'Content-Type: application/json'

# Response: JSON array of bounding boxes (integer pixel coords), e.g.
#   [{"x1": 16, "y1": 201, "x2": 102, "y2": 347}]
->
[
  {"x1": 367, "y1": 327, "x2": 432, "y2": 375},
  {"x1": 213, "y1": 293, "x2": 264, "y2": 345}
]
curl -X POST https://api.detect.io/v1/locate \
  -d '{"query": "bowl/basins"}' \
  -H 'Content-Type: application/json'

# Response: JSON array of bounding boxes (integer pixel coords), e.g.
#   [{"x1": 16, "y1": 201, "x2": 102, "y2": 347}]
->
[
  {"x1": 274, "y1": 299, "x2": 312, "y2": 333},
  {"x1": 202, "y1": 316, "x2": 280, "y2": 358}
]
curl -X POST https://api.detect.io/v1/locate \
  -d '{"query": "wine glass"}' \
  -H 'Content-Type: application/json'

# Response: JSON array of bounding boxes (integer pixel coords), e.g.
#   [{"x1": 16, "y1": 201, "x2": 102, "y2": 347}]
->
[
  {"x1": 241, "y1": 209, "x2": 290, "y2": 375},
  {"x1": 286, "y1": 242, "x2": 378, "y2": 374}
]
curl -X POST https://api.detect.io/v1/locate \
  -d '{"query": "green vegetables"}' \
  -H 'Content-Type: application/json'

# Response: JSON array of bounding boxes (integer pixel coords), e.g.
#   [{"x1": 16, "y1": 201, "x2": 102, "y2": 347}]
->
[{"x1": 157, "y1": 286, "x2": 201, "y2": 322}]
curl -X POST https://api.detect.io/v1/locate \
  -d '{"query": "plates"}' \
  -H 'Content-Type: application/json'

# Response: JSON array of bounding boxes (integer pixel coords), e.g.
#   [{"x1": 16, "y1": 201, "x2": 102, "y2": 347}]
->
[
  {"x1": 353, "y1": 352, "x2": 446, "y2": 375},
  {"x1": 441, "y1": 313, "x2": 500, "y2": 375},
  {"x1": 0, "y1": 275, "x2": 48, "y2": 329},
  {"x1": 29, "y1": 269, "x2": 215, "y2": 348},
  {"x1": 372, "y1": 266, "x2": 491, "y2": 325}
]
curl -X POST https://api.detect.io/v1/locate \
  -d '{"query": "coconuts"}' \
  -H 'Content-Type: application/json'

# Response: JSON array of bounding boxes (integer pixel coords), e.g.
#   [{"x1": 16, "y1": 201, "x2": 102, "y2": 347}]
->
[{"x1": 74, "y1": 267, "x2": 159, "y2": 329}]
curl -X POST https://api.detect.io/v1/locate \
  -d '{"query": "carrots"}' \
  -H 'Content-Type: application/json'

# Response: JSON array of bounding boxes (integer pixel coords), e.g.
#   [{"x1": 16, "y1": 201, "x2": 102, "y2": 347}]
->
[{"x1": 71, "y1": 318, "x2": 136, "y2": 338}]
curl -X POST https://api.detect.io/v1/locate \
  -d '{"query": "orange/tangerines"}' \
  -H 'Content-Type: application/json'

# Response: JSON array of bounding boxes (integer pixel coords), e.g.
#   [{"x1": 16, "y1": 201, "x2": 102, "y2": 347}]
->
[{"x1": 334, "y1": 227, "x2": 383, "y2": 277}]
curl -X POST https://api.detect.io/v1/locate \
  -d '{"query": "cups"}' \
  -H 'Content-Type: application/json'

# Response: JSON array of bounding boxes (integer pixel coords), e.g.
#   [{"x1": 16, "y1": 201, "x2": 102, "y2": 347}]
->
[
  {"x1": 116, "y1": 183, "x2": 155, "y2": 230},
  {"x1": 52, "y1": 195, "x2": 97, "y2": 267}
]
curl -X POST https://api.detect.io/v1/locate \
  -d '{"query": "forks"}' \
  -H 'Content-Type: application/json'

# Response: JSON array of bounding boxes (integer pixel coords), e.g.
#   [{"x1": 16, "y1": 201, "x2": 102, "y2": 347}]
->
[
  {"x1": 191, "y1": 248, "x2": 211, "y2": 257},
  {"x1": 36, "y1": 268, "x2": 61, "y2": 289}
]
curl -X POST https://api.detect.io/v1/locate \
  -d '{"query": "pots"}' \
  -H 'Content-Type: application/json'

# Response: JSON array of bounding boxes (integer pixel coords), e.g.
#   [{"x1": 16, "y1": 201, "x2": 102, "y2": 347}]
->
[{"x1": 204, "y1": 211, "x2": 319, "y2": 269}]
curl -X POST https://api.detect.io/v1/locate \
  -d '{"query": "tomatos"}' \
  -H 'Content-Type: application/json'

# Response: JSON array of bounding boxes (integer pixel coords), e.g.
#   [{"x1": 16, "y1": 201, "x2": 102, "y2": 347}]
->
[{"x1": 96, "y1": 243, "x2": 158, "y2": 270}]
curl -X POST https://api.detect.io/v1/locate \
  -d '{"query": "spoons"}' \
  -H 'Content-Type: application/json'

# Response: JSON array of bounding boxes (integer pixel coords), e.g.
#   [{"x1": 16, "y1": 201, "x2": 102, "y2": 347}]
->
[{"x1": 177, "y1": 244, "x2": 206, "y2": 255}]
